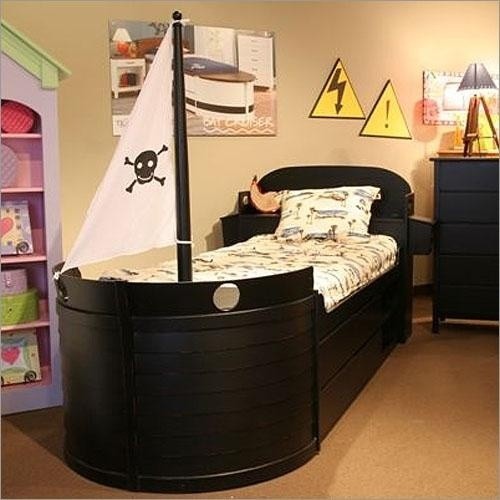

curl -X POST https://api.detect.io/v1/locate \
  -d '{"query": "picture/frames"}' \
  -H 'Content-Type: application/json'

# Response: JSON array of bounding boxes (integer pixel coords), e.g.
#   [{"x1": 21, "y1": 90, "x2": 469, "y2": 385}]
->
[{"x1": 422, "y1": 68, "x2": 500, "y2": 126}]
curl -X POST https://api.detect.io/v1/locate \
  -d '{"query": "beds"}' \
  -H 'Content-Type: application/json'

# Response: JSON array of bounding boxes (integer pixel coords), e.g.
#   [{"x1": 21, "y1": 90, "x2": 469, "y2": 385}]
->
[{"x1": 52, "y1": 166, "x2": 430, "y2": 493}]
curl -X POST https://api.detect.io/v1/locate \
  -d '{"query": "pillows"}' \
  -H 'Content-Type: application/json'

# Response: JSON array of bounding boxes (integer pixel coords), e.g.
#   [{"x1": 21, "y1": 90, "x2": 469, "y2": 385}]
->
[{"x1": 274, "y1": 186, "x2": 380, "y2": 243}]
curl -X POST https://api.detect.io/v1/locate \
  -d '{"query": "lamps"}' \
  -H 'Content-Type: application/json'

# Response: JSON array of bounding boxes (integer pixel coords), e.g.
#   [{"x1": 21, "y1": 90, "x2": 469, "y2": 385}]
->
[{"x1": 456, "y1": 63, "x2": 499, "y2": 157}]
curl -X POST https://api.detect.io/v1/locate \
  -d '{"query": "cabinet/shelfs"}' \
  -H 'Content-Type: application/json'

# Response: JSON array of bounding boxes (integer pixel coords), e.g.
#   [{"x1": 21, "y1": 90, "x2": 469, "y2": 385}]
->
[
  {"x1": 429, "y1": 157, "x2": 499, "y2": 332},
  {"x1": 0, "y1": 15, "x2": 72, "y2": 415}
]
